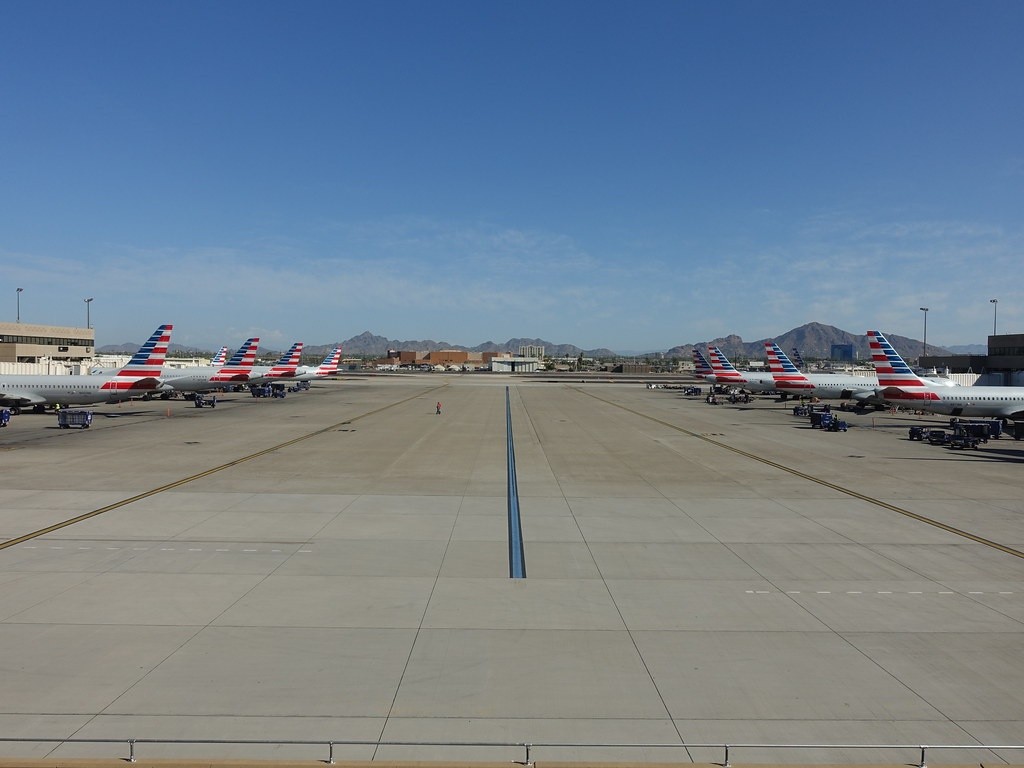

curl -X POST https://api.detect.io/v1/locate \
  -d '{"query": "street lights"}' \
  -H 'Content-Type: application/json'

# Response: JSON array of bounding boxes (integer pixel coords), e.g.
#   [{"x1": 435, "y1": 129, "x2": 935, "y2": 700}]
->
[
  {"x1": 84, "y1": 298, "x2": 94, "y2": 329},
  {"x1": 989, "y1": 298, "x2": 998, "y2": 336},
  {"x1": 920, "y1": 307, "x2": 929, "y2": 355},
  {"x1": 16, "y1": 287, "x2": 24, "y2": 323}
]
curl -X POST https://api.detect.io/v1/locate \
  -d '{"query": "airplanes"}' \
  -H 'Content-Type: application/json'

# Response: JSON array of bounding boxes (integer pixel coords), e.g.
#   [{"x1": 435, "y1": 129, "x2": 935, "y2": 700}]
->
[
  {"x1": 1, "y1": 322, "x2": 175, "y2": 414},
  {"x1": 849, "y1": 330, "x2": 1024, "y2": 428},
  {"x1": 92, "y1": 338, "x2": 345, "y2": 403},
  {"x1": 690, "y1": 341, "x2": 963, "y2": 416}
]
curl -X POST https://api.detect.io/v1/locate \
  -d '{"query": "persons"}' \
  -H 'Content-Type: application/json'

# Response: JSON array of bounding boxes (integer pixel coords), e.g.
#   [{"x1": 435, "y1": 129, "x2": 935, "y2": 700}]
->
[
  {"x1": 213, "y1": 396, "x2": 217, "y2": 404},
  {"x1": 731, "y1": 392, "x2": 749, "y2": 404},
  {"x1": 833, "y1": 414, "x2": 838, "y2": 432},
  {"x1": 436, "y1": 401, "x2": 441, "y2": 414}
]
